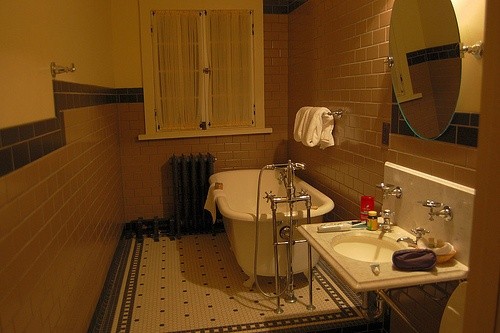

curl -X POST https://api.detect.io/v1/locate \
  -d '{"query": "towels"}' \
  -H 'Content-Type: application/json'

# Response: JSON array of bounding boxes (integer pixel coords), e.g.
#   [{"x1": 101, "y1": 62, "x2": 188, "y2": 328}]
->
[
  {"x1": 204, "y1": 183, "x2": 226, "y2": 223},
  {"x1": 291, "y1": 107, "x2": 335, "y2": 149}
]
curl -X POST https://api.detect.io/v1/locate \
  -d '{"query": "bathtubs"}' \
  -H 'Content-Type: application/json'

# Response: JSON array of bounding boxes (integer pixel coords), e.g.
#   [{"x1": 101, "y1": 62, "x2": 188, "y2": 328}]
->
[{"x1": 210, "y1": 167, "x2": 334, "y2": 286}]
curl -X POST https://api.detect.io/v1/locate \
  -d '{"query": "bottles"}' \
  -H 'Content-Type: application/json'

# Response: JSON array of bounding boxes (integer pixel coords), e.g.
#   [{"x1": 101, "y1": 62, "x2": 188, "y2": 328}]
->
[
  {"x1": 360, "y1": 196, "x2": 374, "y2": 222},
  {"x1": 383, "y1": 209, "x2": 391, "y2": 233},
  {"x1": 368, "y1": 210, "x2": 378, "y2": 230}
]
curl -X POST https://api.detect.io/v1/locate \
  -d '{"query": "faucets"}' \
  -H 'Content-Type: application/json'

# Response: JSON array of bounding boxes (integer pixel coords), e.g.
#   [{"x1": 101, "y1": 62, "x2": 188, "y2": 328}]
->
[
  {"x1": 397, "y1": 227, "x2": 429, "y2": 248},
  {"x1": 378, "y1": 209, "x2": 391, "y2": 231}
]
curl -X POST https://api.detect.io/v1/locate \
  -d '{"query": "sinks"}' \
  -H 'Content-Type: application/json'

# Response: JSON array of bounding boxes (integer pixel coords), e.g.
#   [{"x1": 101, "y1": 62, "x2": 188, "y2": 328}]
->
[{"x1": 332, "y1": 232, "x2": 405, "y2": 262}]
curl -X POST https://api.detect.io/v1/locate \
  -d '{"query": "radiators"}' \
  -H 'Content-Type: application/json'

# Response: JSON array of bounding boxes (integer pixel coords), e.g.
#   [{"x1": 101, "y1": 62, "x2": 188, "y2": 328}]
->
[{"x1": 168, "y1": 151, "x2": 224, "y2": 236}]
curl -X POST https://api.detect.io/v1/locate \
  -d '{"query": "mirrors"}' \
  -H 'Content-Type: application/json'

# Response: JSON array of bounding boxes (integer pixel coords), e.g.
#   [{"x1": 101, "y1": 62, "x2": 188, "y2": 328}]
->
[{"x1": 389, "y1": 0, "x2": 461, "y2": 140}]
